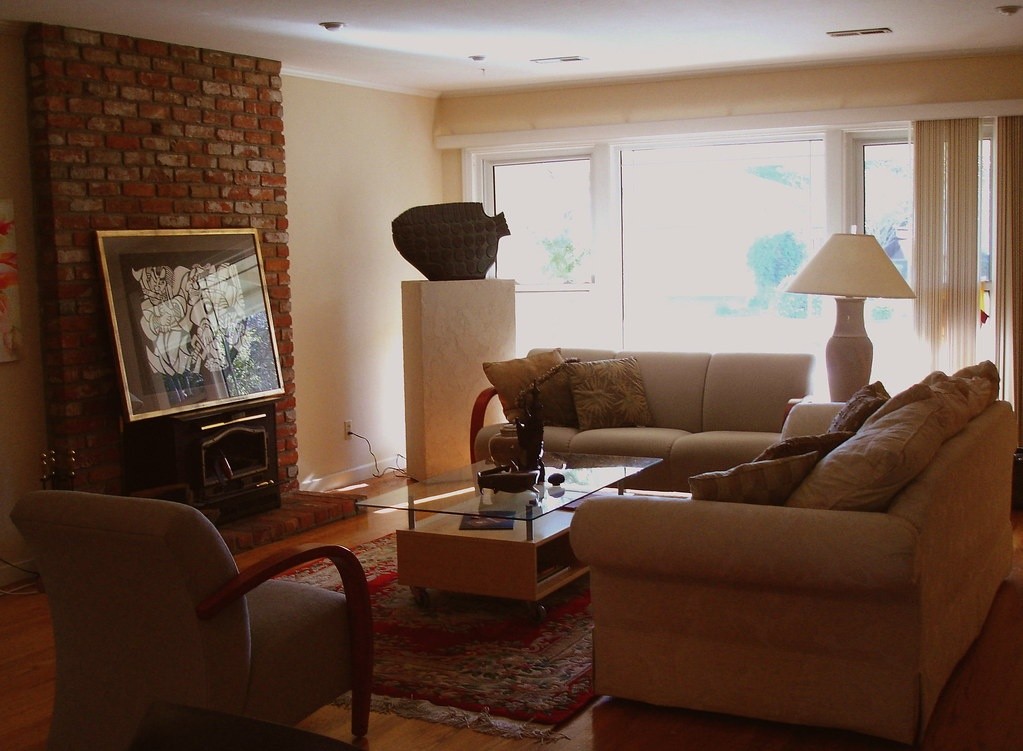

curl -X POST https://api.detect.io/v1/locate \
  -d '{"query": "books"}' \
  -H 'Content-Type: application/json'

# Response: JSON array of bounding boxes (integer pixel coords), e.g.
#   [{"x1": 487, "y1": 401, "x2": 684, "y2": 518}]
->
[{"x1": 459, "y1": 511, "x2": 516, "y2": 530}]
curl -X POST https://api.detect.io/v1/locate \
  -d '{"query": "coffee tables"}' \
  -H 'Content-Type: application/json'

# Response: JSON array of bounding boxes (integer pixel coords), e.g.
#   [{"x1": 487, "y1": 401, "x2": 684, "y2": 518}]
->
[{"x1": 355, "y1": 452, "x2": 662, "y2": 618}]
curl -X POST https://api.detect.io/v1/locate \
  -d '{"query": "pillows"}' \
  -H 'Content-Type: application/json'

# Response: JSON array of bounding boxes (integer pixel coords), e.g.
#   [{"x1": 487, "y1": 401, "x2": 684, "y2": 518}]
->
[
  {"x1": 685, "y1": 360, "x2": 1000, "y2": 512},
  {"x1": 563, "y1": 357, "x2": 656, "y2": 432},
  {"x1": 512, "y1": 355, "x2": 580, "y2": 427},
  {"x1": 482, "y1": 348, "x2": 567, "y2": 424}
]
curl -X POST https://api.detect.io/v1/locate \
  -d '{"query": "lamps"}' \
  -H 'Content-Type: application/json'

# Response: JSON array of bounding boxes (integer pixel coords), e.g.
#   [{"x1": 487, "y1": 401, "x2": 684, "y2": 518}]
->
[{"x1": 785, "y1": 231, "x2": 918, "y2": 401}]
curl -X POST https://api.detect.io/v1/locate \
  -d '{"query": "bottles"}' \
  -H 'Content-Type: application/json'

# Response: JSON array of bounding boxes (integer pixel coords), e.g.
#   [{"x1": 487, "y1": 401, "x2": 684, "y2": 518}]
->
[{"x1": 489, "y1": 423, "x2": 521, "y2": 467}]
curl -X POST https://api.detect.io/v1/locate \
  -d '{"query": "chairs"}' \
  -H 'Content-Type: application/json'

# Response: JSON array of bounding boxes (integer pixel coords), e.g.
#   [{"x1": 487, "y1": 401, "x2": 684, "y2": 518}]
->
[{"x1": 9, "y1": 492, "x2": 371, "y2": 751}]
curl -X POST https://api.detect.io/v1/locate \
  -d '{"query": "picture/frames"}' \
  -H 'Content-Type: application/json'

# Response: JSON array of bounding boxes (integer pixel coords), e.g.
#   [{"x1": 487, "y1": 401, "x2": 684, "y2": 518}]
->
[{"x1": 94, "y1": 229, "x2": 287, "y2": 424}]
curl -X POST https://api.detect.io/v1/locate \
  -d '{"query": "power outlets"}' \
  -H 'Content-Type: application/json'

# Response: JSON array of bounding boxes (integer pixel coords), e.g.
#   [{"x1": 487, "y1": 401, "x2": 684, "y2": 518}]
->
[{"x1": 344, "y1": 421, "x2": 353, "y2": 440}]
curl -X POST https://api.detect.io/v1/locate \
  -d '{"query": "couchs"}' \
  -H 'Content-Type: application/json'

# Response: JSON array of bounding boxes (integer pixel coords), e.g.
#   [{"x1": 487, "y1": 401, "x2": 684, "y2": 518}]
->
[
  {"x1": 567, "y1": 398, "x2": 1016, "y2": 750},
  {"x1": 466, "y1": 346, "x2": 815, "y2": 492}
]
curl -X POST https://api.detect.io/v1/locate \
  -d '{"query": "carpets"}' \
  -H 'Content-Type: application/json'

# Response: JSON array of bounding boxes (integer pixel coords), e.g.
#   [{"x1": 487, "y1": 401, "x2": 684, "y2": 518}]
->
[{"x1": 271, "y1": 529, "x2": 602, "y2": 742}]
